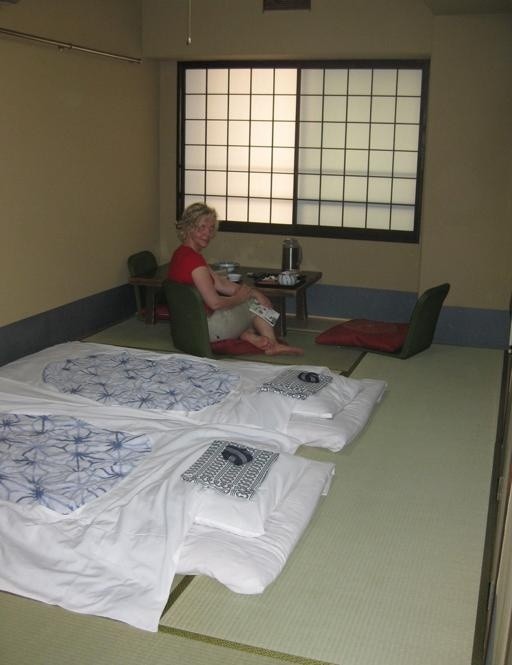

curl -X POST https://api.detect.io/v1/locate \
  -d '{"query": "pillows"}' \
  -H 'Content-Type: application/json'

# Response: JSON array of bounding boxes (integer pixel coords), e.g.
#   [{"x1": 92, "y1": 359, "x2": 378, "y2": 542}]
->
[{"x1": 193, "y1": 370, "x2": 367, "y2": 539}]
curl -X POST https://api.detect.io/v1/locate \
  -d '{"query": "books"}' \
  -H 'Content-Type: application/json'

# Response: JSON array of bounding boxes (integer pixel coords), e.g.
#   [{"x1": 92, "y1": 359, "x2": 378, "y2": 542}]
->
[{"x1": 249, "y1": 298, "x2": 282, "y2": 327}]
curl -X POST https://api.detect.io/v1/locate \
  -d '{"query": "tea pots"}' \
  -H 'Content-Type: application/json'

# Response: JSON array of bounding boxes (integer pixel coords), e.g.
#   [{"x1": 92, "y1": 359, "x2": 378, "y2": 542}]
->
[{"x1": 277, "y1": 270, "x2": 302, "y2": 286}]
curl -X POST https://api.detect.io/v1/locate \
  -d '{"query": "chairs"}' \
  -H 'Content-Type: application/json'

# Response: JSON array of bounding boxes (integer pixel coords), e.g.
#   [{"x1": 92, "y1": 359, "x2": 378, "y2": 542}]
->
[
  {"x1": 316, "y1": 282, "x2": 452, "y2": 360},
  {"x1": 126, "y1": 250, "x2": 288, "y2": 360}
]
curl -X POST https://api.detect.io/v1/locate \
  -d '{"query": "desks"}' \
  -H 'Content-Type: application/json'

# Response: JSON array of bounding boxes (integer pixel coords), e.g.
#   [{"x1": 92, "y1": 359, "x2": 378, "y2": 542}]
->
[{"x1": 129, "y1": 262, "x2": 324, "y2": 337}]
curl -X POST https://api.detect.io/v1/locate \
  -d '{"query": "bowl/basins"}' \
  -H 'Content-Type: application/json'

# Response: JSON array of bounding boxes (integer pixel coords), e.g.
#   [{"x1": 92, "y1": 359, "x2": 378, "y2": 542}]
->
[
  {"x1": 215, "y1": 261, "x2": 240, "y2": 273},
  {"x1": 227, "y1": 273, "x2": 242, "y2": 282}
]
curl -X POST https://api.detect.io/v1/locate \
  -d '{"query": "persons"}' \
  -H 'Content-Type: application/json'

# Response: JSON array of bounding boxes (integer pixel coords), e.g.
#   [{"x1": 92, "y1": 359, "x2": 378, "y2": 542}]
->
[{"x1": 166, "y1": 202, "x2": 306, "y2": 357}]
